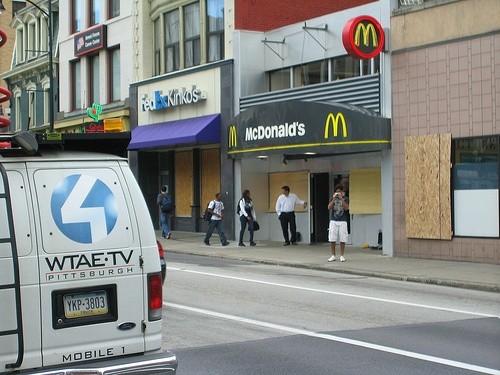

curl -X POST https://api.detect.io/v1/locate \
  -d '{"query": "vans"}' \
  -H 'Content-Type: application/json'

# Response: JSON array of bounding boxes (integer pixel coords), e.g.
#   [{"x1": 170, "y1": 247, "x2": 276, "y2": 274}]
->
[{"x1": 0, "y1": 121, "x2": 179, "y2": 375}]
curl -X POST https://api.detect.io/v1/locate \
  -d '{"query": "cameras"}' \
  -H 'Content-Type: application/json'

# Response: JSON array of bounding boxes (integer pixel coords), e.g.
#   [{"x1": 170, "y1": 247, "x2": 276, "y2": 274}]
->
[{"x1": 336, "y1": 193, "x2": 339, "y2": 197}]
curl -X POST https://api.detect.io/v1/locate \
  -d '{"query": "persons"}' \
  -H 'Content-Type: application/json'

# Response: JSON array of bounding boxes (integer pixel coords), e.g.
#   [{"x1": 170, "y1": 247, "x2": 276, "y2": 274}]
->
[
  {"x1": 157, "y1": 187, "x2": 174, "y2": 239},
  {"x1": 275, "y1": 185, "x2": 307, "y2": 246},
  {"x1": 328, "y1": 185, "x2": 349, "y2": 262},
  {"x1": 204, "y1": 193, "x2": 229, "y2": 246},
  {"x1": 237, "y1": 190, "x2": 257, "y2": 247}
]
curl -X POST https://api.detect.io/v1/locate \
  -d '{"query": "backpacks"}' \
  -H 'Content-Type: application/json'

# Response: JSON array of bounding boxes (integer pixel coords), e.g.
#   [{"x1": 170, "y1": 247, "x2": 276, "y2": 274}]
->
[
  {"x1": 202, "y1": 200, "x2": 216, "y2": 221},
  {"x1": 160, "y1": 194, "x2": 172, "y2": 212},
  {"x1": 236, "y1": 197, "x2": 242, "y2": 215}
]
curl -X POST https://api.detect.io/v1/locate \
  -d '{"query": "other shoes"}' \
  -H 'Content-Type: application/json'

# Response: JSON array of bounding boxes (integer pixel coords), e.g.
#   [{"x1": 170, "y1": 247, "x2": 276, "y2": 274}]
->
[
  {"x1": 222, "y1": 240, "x2": 231, "y2": 246},
  {"x1": 328, "y1": 255, "x2": 336, "y2": 262},
  {"x1": 340, "y1": 256, "x2": 345, "y2": 262},
  {"x1": 292, "y1": 242, "x2": 298, "y2": 245},
  {"x1": 238, "y1": 242, "x2": 245, "y2": 246},
  {"x1": 250, "y1": 241, "x2": 256, "y2": 246},
  {"x1": 283, "y1": 242, "x2": 290, "y2": 246},
  {"x1": 166, "y1": 232, "x2": 171, "y2": 239},
  {"x1": 161, "y1": 235, "x2": 166, "y2": 239},
  {"x1": 203, "y1": 238, "x2": 211, "y2": 246}
]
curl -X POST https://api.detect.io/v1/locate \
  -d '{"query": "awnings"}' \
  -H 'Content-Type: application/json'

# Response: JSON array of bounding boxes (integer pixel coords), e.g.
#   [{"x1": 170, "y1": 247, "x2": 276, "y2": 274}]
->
[
  {"x1": 227, "y1": 99, "x2": 392, "y2": 160},
  {"x1": 127, "y1": 114, "x2": 221, "y2": 150}
]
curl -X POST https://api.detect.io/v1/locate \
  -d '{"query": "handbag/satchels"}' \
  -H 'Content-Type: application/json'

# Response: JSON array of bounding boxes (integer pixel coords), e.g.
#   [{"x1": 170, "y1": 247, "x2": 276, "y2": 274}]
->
[{"x1": 248, "y1": 221, "x2": 259, "y2": 231}]
